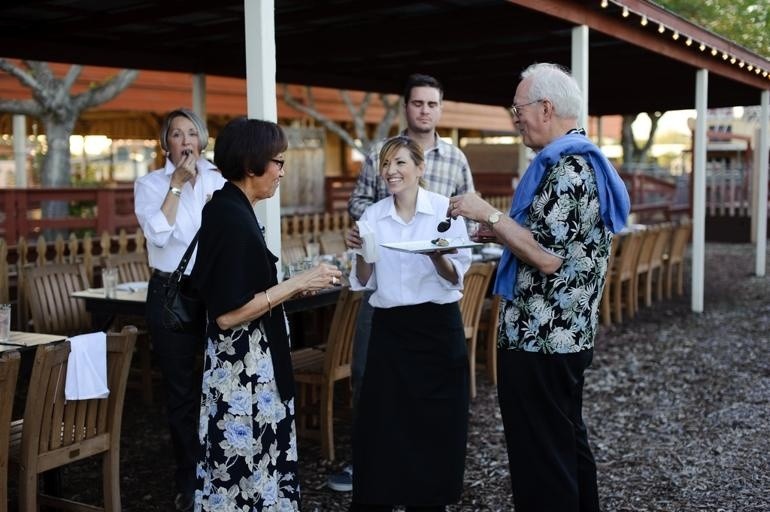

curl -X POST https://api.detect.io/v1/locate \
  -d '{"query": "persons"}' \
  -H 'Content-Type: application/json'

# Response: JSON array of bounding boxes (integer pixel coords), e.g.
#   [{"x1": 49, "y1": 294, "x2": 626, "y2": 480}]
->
[
  {"x1": 447, "y1": 65, "x2": 612, "y2": 512},
  {"x1": 344, "y1": 137, "x2": 473, "y2": 512},
  {"x1": 192, "y1": 116, "x2": 342, "y2": 511},
  {"x1": 134, "y1": 108, "x2": 233, "y2": 512},
  {"x1": 330, "y1": 75, "x2": 480, "y2": 491}
]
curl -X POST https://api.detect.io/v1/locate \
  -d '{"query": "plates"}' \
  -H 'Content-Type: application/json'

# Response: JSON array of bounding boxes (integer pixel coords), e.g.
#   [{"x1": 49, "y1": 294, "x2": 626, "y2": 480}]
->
[{"x1": 380, "y1": 238, "x2": 484, "y2": 254}]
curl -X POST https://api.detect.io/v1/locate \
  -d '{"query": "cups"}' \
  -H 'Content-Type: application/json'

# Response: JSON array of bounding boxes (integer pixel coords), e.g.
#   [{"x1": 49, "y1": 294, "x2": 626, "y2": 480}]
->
[
  {"x1": 306, "y1": 243, "x2": 320, "y2": 257},
  {"x1": 289, "y1": 261, "x2": 304, "y2": 276},
  {"x1": 102, "y1": 268, "x2": 118, "y2": 299}
]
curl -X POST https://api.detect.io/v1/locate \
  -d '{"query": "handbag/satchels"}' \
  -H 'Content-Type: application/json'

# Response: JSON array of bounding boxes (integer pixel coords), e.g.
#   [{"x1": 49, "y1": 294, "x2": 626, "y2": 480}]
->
[{"x1": 162, "y1": 223, "x2": 205, "y2": 359}]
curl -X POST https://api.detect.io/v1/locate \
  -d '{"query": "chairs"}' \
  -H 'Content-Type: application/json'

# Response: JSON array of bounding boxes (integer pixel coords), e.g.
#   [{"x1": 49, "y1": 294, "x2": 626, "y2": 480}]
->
[
  {"x1": 612, "y1": 234, "x2": 633, "y2": 323},
  {"x1": 625, "y1": 229, "x2": 645, "y2": 319},
  {"x1": 651, "y1": 224, "x2": 676, "y2": 309},
  {"x1": 666, "y1": 224, "x2": 692, "y2": 299},
  {"x1": 603, "y1": 234, "x2": 614, "y2": 331},
  {"x1": 281, "y1": 237, "x2": 307, "y2": 266},
  {"x1": 292, "y1": 284, "x2": 364, "y2": 462},
  {"x1": 102, "y1": 253, "x2": 151, "y2": 285},
  {"x1": 8, "y1": 325, "x2": 138, "y2": 511},
  {"x1": 639, "y1": 226, "x2": 659, "y2": 316},
  {"x1": 21, "y1": 262, "x2": 113, "y2": 338},
  {"x1": 319, "y1": 231, "x2": 350, "y2": 256},
  {"x1": 0, "y1": 349, "x2": 20, "y2": 512},
  {"x1": 459, "y1": 262, "x2": 497, "y2": 401}
]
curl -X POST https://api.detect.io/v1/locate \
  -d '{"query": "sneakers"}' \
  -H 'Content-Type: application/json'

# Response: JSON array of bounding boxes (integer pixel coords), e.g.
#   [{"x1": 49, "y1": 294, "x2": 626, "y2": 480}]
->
[{"x1": 327, "y1": 464, "x2": 353, "y2": 491}]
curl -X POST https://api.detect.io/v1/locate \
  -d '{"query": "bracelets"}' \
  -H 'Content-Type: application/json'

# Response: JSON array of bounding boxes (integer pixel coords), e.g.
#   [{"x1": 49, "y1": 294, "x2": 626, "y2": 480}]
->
[
  {"x1": 264, "y1": 289, "x2": 278, "y2": 316},
  {"x1": 168, "y1": 187, "x2": 182, "y2": 199}
]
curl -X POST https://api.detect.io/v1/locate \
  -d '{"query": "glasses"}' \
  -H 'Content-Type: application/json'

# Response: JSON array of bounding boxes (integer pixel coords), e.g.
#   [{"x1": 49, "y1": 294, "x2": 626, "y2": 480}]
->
[
  {"x1": 510, "y1": 98, "x2": 543, "y2": 116},
  {"x1": 269, "y1": 159, "x2": 284, "y2": 169}
]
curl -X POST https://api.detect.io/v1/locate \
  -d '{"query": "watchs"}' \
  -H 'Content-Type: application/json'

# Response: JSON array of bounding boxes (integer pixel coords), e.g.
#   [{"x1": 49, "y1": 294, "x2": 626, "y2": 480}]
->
[{"x1": 487, "y1": 209, "x2": 504, "y2": 232}]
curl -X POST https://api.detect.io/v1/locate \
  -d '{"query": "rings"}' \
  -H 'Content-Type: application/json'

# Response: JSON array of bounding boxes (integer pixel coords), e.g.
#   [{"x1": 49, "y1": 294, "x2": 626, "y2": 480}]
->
[
  {"x1": 453, "y1": 203, "x2": 458, "y2": 209},
  {"x1": 332, "y1": 276, "x2": 339, "y2": 285}
]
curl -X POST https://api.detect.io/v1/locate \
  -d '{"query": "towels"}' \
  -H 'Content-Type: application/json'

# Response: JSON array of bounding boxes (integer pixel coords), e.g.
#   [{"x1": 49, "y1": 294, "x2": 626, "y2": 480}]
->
[{"x1": 54, "y1": 330, "x2": 110, "y2": 405}]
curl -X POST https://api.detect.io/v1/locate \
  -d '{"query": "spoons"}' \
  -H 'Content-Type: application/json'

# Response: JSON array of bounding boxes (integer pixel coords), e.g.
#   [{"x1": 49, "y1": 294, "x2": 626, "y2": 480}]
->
[{"x1": 437, "y1": 192, "x2": 454, "y2": 233}]
